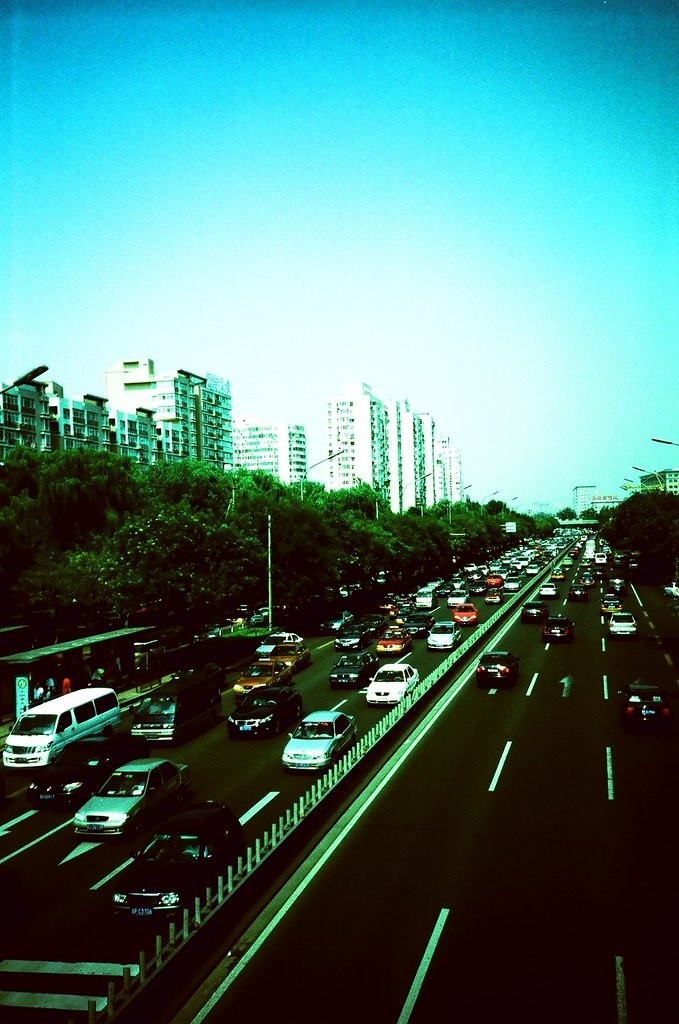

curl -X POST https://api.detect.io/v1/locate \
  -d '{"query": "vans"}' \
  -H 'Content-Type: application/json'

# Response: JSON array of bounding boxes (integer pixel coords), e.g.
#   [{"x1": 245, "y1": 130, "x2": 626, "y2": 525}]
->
[
  {"x1": 3, "y1": 687, "x2": 122, "y2": 768},
  {"x1": 130, "y1": 677, "x2": 222, "y2": 742},
  {"x1": 415, "y1": 586, "x2": 438, "y2": 609}
]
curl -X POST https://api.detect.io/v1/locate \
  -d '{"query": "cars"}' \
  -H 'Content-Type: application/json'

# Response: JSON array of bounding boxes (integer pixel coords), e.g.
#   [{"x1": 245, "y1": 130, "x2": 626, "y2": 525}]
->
[
  {"x1": 600, "y1": 594, "x2": 622, "y2": 615},
  {"x1": 359, "y1": 614, "x2": 389, "y2": 639},
  {"x1": 447, "y1": 590, "x2": 471, "y2": 607},
  {"x1": 395, "y1": 604, "x2": 416, "y2": 626},
  {"x1": 608, "y1": 612, "x2": 638, "y2": 635},
  {"x1": 27, "y1": 733, "x2": 150, "y2": 809},
  {"x1": 403, "y1": 612, "x2": 435, "y2": 637},
  {"x1": 228, "y1": 684, "x2": 303, "y2": 738},
  {"x1": 255, "y1": 632, "x2": 304, "y2": 657},
  {"x1": 542, "y1": 616, "x2": 576, "y2": 642},
  {"x1": 282, "y1": 710, "x2": 358, "y2": 770},
  {"x1": 134, "y1": 604, "x2": 268, "y2": 658},
  {"x1": 485, "y1": 588, "x2": 504, "y2": 604},
  {"x1": 233, "y1": 657, "x2": 293, "y2": 697},
  {"x1": 664, "y1": 582, "x2": 679, "y2": 599},
  {"x1": 389, "y1": 598, "x2": 414, "y2": 617},
  {"x1": 110, "y1": 801, "x2": 245, "y2": 921},
  {"x1": 378, "y1": 593, "x2": 401, "y2": 609},
  {"x1": 268, "y1": 642, "x2": 310, "y2": 673},
  {"x1": 376, "y1": 625, "x2": 414, "y2": 656},
  {"x1": 320, "y1": 611, "x2": 354, "y2": 632},
  {"x1": 330, "y1": 652, "x2": 380, "y2": 684},
  {"x1": 73, "y1": 758, "x2": 196, "y2": 836},
  {"x1": 339, "y1": 529, "x2": 639, "y2": 599},
  {"x1": 335, "y1": 624, "x2": 372, "y2": 651},
  {"x1": 617, "y1": 683, "x2": 670, "y2": 728},
  {"x1": 452, "y1": 603, "x2": 478, "y2": 627},
  {"x1": 366, "y1": 663, "x2": 419, "y2": 704},
  {"x1": 427, "y1": 621, "x2": 462, "y2": 650},
  {"x1": 475, "y1": 651, "x2": 519, "y2": 687},
  {"x1": 522, "y1": 601, "x2": 549, "y2": 622}
]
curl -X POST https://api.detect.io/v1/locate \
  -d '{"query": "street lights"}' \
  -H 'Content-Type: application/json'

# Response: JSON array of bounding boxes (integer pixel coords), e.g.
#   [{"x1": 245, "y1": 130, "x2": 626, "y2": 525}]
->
[
  {"x1": 449, "y1": 484, "x2": 472, "y2": 524},
  {"x1": 481, "y1": 490, "x2": 499, "y2": 514},
  {"x1": 399, "y1": 472, "x2": 431, "y2": 515},
  {"x1": 624, "y1": 478, "x2": 650, "y2": 493},
  {"x1": 633, "y1": 466, "x2": 667, "y2": 494},
  {"x1": 502, "y1": 496, "x2": 518, "y2": 519},
  {"x1": 620, "y1": 486, "x2": 634, "y2": 494},
  {"x1": 623, "y1": 485, "x2": 637, "y2": 492},
  {"x1": 300, "y1": 449, "x2": 345, "y2": 506}
]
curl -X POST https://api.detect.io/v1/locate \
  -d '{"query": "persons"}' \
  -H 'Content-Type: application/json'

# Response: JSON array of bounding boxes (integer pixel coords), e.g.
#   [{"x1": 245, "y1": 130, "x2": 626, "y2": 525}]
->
[{"x1": 26, "y1": 663, "x2": 103, "y2": 696}]
